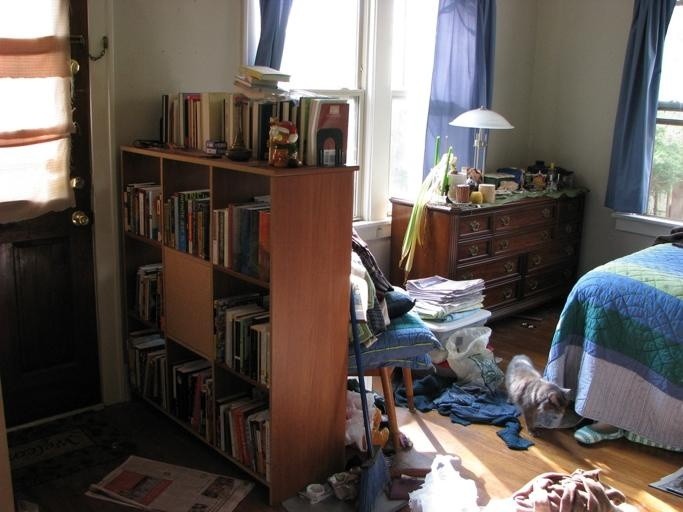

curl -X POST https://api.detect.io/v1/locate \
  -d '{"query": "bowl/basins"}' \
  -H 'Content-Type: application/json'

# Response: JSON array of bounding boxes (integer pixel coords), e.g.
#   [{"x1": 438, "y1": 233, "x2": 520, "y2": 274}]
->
[{"x1": 224, "y1": 148, "x2": 252, "y2": 162}]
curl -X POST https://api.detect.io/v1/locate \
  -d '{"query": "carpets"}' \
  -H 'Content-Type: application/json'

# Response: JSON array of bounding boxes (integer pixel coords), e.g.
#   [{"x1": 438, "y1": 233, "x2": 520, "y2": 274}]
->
[{"x1": 7, "y1": 409, "x2": 137, "y2": 492}]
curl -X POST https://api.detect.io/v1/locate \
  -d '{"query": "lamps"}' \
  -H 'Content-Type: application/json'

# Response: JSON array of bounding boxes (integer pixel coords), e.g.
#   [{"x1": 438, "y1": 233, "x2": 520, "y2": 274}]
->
[{"x1": 448, "y1": 105, "x2": 515, "y2": 184}]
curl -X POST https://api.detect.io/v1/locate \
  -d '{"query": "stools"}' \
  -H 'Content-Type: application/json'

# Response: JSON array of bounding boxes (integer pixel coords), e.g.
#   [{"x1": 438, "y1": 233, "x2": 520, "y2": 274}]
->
[{"x1": 347, "y1": 365, "x2": 415, "y2": 450}]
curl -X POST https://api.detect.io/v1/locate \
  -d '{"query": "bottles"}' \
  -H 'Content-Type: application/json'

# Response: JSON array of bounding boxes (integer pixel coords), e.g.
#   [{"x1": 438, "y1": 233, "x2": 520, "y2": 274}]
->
[{"x1": 546, "y1": 162, "x2": 558, "y2": 194}]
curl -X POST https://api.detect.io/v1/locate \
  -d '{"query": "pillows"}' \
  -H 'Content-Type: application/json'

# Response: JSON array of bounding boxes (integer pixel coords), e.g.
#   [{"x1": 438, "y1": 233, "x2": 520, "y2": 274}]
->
[{"x1": 348, "y1": 286, "x2": 438, "y2": 369}]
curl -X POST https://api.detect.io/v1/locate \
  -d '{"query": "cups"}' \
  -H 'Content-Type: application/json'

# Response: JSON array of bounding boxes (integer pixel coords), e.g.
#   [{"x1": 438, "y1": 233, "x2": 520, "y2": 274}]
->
[{"x1": 447, "y1": 173, "x2": 496, "y2": 203}]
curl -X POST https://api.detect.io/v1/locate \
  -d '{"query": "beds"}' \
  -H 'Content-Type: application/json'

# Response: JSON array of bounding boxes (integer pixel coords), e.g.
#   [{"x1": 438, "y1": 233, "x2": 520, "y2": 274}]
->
[{"x1": 577, "y1": 243, "x2": 683, "y2": 452}]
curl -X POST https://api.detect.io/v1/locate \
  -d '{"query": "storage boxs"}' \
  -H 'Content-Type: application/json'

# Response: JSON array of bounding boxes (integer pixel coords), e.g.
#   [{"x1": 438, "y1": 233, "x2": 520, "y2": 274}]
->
[{"x1": 420, "y1": 310, "x2": 490, "y2": 362}]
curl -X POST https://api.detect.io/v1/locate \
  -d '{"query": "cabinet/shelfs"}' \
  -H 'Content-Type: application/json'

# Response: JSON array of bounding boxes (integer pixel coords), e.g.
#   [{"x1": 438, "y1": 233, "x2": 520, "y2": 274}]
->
[
  {"x1": 389, "y1": 196, "x2": 587, "y2": 329},
  {"x1": 118, "y1": 143, "x2": 359, "y2": 507}
]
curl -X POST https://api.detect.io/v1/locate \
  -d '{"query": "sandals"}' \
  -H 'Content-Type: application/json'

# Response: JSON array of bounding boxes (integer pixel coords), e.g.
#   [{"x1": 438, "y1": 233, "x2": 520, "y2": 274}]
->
[
  {"x1": 573, "y1": 422, "x2": 626, "y2": 446},
  {"x1": 620, "y1": 427, "x2": 682, "y2": 453}
]
